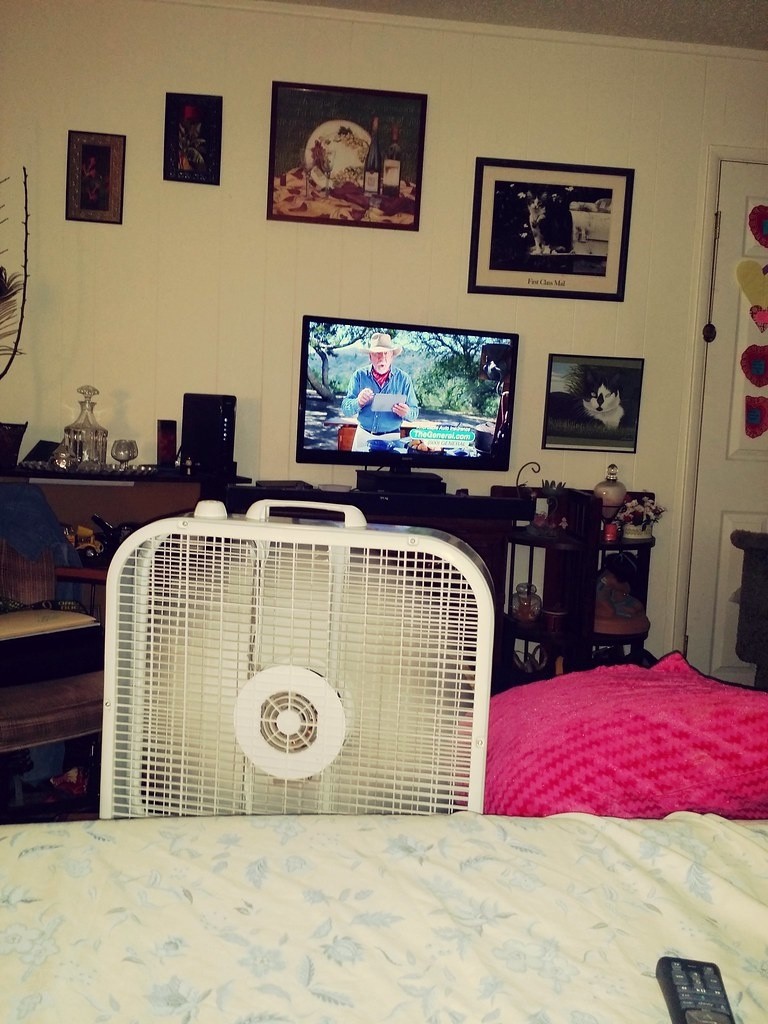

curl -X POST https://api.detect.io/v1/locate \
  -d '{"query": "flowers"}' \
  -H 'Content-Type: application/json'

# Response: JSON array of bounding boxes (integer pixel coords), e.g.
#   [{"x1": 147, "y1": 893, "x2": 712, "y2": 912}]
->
[{"x1": 617, "y1": 493, "x2": 665, "y2": 531}]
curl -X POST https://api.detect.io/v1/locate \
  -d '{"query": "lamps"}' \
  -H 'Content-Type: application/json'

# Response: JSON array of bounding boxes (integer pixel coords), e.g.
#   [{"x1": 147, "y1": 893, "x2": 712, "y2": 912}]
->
[{"x1": 593, "y1": 463, "x2": 627, "y2": 526}]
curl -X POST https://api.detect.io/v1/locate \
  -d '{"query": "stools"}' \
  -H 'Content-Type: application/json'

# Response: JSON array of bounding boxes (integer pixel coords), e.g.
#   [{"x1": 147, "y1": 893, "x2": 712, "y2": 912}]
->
[{"x1": 454, "y1": 651, "x2": 768, "y2": 819}]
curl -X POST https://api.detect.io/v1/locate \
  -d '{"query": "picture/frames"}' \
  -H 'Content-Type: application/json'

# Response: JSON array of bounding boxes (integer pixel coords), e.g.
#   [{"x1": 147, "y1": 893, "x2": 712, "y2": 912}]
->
[
  {"x1": 542, "y1": 351, "x2": 645, "y2": 453},
  {"x1": 267, "y1": 79, "x2": 428, "y2": 233},
  {"x1": 162, "y1": 90, "x2": 223, "y2": 186},
  {"x1": 467, "y1": 156, "x2": 636, "y2": 302},
  {"x1": 66, "y1": 131, "x2": 128, "y2": 225}
]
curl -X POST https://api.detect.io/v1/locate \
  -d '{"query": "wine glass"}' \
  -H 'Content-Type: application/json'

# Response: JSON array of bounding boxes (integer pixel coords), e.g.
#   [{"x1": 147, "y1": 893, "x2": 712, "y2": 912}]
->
[{"x1": 111, "y1": 440, "x2": 139, "y2": 471}]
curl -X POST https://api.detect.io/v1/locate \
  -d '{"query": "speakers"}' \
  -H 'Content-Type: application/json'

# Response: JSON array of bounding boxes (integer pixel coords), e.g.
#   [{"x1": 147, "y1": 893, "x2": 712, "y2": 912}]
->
[{"x1": 180, "y1": 393, "x2": 238, "y2": 480}]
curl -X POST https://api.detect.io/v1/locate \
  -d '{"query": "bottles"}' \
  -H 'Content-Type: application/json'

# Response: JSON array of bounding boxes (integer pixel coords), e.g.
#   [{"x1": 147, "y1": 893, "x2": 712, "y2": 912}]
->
[
  {"x1": 512, "y1": 584, "x2": 543, "y2": 622},
  {"x1": 92, "y1": 513, "x2": 114, "y2": 535},
  {"x1": 64, "y1": 385, "x2": 108, "y2": 465}
]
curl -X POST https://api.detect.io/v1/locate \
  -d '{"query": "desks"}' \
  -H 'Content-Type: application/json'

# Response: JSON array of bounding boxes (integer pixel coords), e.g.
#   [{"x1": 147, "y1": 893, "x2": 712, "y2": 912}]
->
[
  {"x1": 0, "y1": 472, "x2": 252, "y2": 624},
  {"x1": 324, "y1": 417, "x2": 443, "y2": 450},
  {"x1": 233, "y1": 486, "x2": 508, "y2": 616}
]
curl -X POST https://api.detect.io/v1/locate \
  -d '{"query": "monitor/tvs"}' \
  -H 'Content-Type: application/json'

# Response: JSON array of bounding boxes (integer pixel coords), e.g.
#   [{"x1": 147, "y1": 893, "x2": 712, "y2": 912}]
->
[{"x1": 296, "y1": 315, "x2": 519, "y2": 474}]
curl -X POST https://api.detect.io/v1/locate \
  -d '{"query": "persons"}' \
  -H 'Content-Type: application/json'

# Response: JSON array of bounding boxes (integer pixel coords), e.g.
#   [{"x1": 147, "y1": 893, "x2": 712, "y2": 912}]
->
[{"x1": 341, "y1": 333, "x2": 420, "y2": 453}]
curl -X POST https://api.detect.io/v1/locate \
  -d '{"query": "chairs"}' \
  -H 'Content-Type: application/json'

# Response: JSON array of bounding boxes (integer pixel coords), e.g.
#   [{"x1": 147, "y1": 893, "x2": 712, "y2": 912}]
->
[
  {"x1": 731, "y1": 529, "x2": 768, "y2": 689},
  {"x1": 0, "y1": 484, "x2": 105, "y2": 752}
]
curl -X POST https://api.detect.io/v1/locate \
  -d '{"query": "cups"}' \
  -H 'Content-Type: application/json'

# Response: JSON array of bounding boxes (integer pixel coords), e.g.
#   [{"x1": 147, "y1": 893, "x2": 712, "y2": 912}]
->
[{"x1": 76, "y1": 526, "x2": 93, "y2": 539}]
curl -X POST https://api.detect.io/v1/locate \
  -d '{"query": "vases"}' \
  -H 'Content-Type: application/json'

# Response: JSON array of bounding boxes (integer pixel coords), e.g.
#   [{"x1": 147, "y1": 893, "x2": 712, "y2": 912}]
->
[{"x1": 623, "y1": 524, "x2": 654, "y2": 538}]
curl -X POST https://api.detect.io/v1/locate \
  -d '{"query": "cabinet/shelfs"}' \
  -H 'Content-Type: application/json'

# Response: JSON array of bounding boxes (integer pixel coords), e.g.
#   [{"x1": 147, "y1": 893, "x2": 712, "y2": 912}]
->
[{"x1": 492, "y1": 486, "x2": 652, "y2": 678}]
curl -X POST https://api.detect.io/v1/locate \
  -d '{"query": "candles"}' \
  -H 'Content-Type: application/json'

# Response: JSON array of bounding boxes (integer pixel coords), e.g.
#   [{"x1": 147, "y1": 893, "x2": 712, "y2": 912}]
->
[{"x1": 604, "y1": 524, "x2": 616, "y2": 541}]
